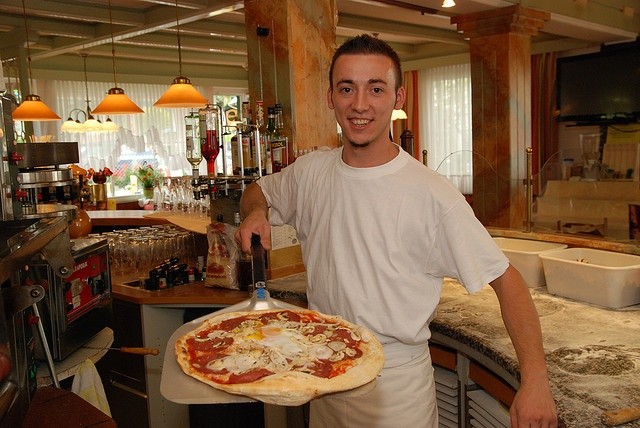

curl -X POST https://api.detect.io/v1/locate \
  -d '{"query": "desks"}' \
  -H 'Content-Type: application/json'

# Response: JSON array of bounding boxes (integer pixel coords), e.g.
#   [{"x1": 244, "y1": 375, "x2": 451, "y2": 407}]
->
[{"x1": 28, "y1": 327, "x2": 116, "y2": 386}]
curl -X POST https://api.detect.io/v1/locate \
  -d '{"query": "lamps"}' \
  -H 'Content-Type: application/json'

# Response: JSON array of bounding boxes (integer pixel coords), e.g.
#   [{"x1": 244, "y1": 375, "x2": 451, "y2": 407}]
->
[
  {"x1": 90, "y1": 1, "x2": 146, "y2": 115},
  {"x1": 12, "y1": 0, "x2": 63, "y2": 122},
  {"x1": 151, "y1": 0, "x2": 209, "y2": 110},
  {"x1": 60, "y1": 49, "x2": 120, "y2": 134}
]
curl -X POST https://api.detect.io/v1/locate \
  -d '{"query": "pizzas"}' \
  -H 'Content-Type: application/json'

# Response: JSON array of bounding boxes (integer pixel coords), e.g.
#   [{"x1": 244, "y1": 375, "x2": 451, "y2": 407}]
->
[{"x1": 174, "y1": 309, "x2": 384, "y2": 406}]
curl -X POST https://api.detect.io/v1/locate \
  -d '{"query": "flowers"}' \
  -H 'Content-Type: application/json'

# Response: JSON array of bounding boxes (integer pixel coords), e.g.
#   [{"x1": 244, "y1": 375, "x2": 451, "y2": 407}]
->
[{"x1": 131, "y1": 161, "x2": 165, "y2": 188}]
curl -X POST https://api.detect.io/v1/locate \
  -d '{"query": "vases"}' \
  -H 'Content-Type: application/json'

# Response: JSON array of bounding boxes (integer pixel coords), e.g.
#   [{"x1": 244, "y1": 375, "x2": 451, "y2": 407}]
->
[{"x1": 143, "y1": 186, "x2": 154, "y2": 199}]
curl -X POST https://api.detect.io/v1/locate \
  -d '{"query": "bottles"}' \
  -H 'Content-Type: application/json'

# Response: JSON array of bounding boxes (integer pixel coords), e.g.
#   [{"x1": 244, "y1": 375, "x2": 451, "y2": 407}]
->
[
  {"x1": 198, "y1": 109, "x2": 219, "y2": 178},
  {"x1": 232, "y1": 212, "x2": 242, "y2": 227},
  {"x1": 239, "y1": 102, "x2": 252, "y2": 138},
  {"x1": 270, "y1": 104, "x2": 289, "y2": 171},
  {"x1": 185, "y1": 115, "x2": 203, "y2": 178},
  {"x1": 231, "y1": 123, "x2": 251, "y2": 174},
  {"x1": 252, "y1": 100, "x2": 268, "y2": 176},
  {"x1": 153, "y1": 179, "x2": 212, "y2": 220}
]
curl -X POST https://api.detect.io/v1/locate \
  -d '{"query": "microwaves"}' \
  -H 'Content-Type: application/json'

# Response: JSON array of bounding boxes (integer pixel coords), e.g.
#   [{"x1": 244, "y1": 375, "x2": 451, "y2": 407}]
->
[{"x1": 17, "y1": 237, "x2": 115, "y2": 362}]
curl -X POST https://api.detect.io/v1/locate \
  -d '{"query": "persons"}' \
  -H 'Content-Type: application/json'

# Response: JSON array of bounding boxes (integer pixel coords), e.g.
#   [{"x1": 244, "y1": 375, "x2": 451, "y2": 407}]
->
[{"x1": 233, "y1": 35, "x2": 558, "y2": 428}]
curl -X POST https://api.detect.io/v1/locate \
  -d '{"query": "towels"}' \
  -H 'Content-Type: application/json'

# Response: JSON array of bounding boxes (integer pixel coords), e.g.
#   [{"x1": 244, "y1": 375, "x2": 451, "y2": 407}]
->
[{"x1": 70, "y1": 358, "x2": 112, "y2": 418}]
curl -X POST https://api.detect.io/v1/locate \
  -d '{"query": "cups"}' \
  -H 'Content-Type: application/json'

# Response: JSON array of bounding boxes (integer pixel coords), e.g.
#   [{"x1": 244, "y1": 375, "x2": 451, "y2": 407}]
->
[{"x1": 89, "y1": 224, "x2": 191, "y2": 275}]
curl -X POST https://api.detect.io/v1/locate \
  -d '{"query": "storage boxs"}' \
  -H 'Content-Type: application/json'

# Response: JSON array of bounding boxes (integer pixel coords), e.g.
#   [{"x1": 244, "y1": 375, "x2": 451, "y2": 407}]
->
[
  {"x1": 539, "y1": 247, "x2": 639, "y2": 309},
  {"x1": 492, "y1": 237, "x2": 568, "y2": 287}
]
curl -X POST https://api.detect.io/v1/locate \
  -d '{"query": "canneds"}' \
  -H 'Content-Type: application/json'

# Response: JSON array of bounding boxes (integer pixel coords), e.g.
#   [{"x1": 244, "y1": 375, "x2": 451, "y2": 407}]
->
[{"x1": 562, "y1": 158, "x2": 573, "y2": 181}]
